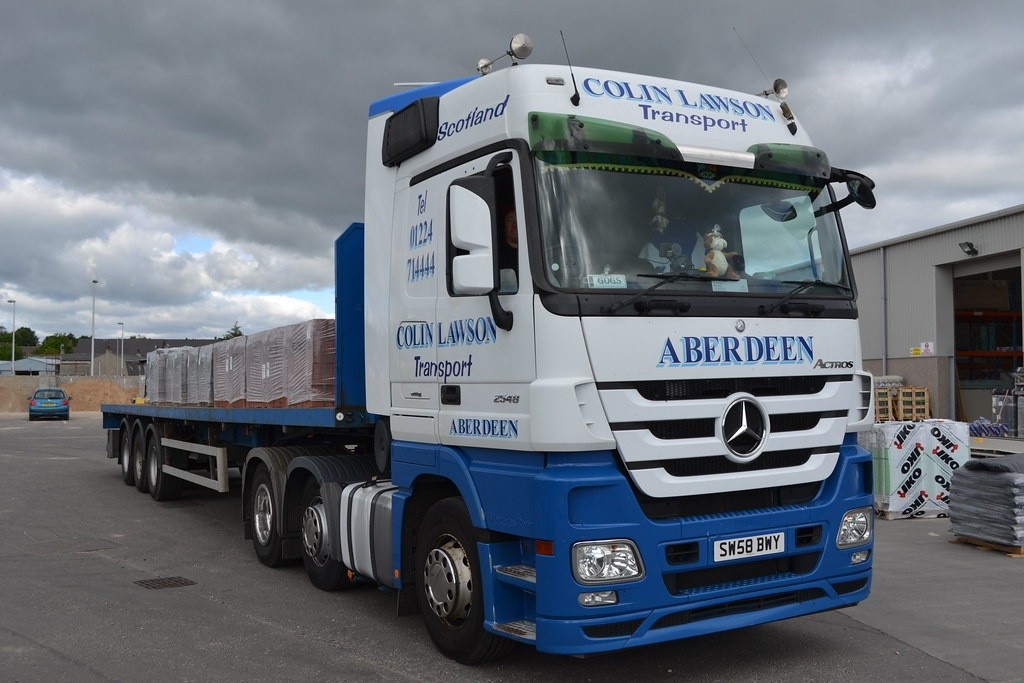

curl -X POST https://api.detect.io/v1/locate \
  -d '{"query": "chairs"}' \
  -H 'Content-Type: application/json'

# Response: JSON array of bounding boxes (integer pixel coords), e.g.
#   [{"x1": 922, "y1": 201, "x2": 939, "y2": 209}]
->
[
  {"x1": 40, "y1": 393, "x2": 46, "y2": 398},
  {"x1": 54, "y1": 391, "x2": 61, "y2": 397}
]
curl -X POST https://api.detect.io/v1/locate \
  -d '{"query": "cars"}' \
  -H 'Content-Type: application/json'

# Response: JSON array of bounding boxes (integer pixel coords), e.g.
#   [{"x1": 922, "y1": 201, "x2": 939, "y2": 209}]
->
[{"x1": 26, "y1": 386, "x2": 73, "y2": 420}]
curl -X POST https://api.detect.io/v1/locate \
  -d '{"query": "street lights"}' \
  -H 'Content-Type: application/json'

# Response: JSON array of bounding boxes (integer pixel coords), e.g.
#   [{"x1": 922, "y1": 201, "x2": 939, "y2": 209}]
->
[
  {"x1": 117, "y1": 323, "x2": 124, "y2": 377},
  {"x1": 7, "y1": 299, "x2": 16, "y2": 375},
  {"x1": 91, "y1": 279, "x2": 99, "y2": 376}
]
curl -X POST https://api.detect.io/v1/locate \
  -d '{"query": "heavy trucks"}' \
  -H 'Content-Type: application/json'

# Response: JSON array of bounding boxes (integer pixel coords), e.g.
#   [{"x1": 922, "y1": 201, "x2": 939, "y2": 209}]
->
[{"x1": 101, "y1": 28, "x2": 886, "y2": 665}]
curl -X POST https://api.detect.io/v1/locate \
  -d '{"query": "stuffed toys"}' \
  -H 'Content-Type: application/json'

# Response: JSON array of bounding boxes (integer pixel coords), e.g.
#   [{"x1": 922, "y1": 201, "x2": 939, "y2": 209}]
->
[{"x1": 703, "y1": 229, "x2": 740, "y2": 280}]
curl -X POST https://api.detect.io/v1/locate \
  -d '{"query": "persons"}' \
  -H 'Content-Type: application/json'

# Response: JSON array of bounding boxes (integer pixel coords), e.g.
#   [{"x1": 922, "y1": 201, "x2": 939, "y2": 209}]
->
[{"x1": 497, "y1": 207, "x2": 518, "y2": 277}]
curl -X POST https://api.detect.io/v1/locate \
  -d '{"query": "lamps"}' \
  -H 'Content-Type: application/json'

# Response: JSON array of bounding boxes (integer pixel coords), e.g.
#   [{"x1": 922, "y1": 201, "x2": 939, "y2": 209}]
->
[{"x1": 959, "y1": 241, "x2": 978, "y2": 256}]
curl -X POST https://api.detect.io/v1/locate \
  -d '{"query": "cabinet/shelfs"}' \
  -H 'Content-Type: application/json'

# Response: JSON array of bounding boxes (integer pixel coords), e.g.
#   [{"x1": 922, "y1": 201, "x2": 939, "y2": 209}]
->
[{"x1": 955, "y1": 310, "x2": 1022, "y2": 389}]
